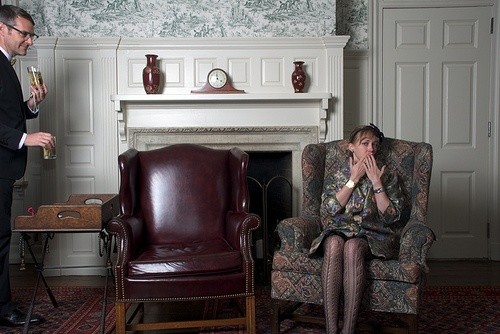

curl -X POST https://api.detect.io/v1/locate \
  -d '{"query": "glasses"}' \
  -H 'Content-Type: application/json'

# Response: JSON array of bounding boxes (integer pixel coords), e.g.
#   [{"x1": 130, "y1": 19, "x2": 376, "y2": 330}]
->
[{"x1": 2, "y1": 21, "x2": 39, "y2": 41}]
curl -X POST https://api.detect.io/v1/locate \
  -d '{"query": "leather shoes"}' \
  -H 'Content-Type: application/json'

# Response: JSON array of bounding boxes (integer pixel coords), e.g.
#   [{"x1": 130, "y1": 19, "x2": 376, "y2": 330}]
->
[{"x1": 0, "y1": 307, "x2": 44, "y2": 327}]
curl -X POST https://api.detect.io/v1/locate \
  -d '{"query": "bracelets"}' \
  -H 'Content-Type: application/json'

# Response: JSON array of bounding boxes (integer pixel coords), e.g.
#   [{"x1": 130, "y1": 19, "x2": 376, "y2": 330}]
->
[
  {"x1": 345, "y1": 179, "x2": 356, "y2": 190},
  {"x1": 373, "y1": 187, "x2": 385, "y2": 194}
]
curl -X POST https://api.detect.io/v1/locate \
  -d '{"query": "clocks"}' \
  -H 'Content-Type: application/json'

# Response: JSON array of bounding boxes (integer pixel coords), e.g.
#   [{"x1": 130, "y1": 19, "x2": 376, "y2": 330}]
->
[{"x1": 191, "y1": 68, "x2": 246, "y2": 94}]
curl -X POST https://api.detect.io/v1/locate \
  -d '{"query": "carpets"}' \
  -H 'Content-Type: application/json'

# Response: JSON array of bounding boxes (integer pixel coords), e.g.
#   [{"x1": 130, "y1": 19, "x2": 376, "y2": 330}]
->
[
  {"x1": 0, "y1": 285, "x2": 132, "y2": 334},
  {"x1": 199, "y1": 285, "x2": 500, "y2": 334}
]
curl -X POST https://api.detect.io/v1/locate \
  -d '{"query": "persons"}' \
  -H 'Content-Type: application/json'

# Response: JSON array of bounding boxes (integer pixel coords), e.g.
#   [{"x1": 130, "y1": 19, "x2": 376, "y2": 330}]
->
[
  {"x1": 0, "y1": 5, "x2": 56, "y2": 327},
  {"x1": 309, "y1": 123, "x2": 403, "y2": 334}
]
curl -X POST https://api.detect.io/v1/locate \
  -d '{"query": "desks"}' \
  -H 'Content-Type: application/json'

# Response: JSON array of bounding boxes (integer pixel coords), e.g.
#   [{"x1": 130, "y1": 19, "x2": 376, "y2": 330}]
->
[{"x1": 11, "y1": 194, "x2": 120, "y2": 334}]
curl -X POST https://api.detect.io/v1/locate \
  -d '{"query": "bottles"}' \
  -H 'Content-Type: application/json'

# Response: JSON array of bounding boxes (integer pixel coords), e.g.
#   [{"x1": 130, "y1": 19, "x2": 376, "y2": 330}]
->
[
  {"x1": 143, "y1": 54, "x2": 161, "y2": 94},
  {"x1": 291, "y1": 61, "x2": 307, "y2": 93}
]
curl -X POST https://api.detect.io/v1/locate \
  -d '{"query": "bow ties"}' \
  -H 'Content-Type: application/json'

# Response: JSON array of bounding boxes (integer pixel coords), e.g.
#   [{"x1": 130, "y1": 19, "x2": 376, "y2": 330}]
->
[{"x1": 10, "y1": 59, "x2": 16, "y2": 66}]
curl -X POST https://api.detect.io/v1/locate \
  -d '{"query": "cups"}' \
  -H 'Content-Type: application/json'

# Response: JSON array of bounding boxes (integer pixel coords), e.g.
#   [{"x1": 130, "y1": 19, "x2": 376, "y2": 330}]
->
[
  {"x1": 43, "y1": 137, "x2": 57, "y2": 160},
  {"x1": 27, "y1": 64, "x2": 43, "y2": 88}
]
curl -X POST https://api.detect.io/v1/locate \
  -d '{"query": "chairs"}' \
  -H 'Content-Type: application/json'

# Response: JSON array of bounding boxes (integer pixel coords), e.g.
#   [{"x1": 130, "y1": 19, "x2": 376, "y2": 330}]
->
[
  {"x1": 107, "y1": 143, "x2": 261, "y2": 334},
  {"x1": 271, "y1": 139, "x2": 435, "y2": 334}
]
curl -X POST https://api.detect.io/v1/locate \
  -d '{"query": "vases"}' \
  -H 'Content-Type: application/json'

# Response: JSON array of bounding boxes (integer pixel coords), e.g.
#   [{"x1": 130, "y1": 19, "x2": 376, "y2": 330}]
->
[
  {"x1": 292, "y1": 61, "x2": 306, "y2": 93},
  {"x1": 143, "y1": 55, "x2": 160, "y2": 94}
]
